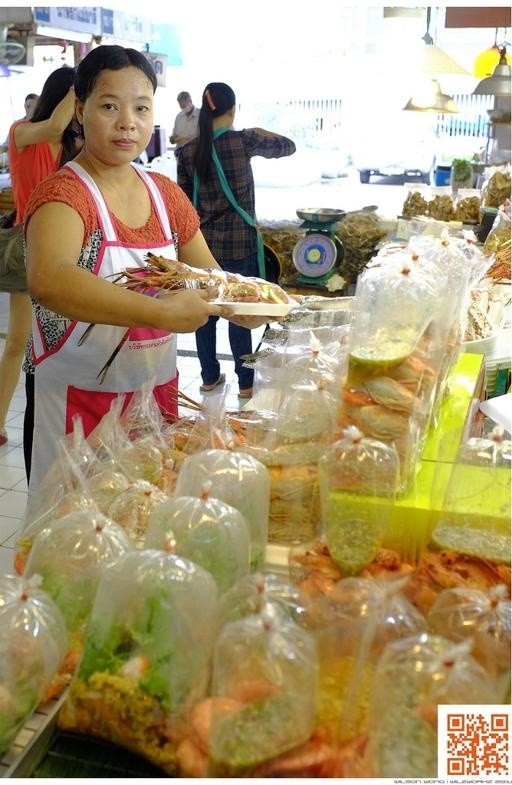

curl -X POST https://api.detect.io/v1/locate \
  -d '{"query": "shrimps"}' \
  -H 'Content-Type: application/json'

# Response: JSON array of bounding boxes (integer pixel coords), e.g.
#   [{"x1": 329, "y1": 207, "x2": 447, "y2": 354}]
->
[
  {"x1": 163, "y1": 369, "x2": 331, "y2": 448},
  {"x1": 177, "y1": 696, "x2": 368, "y2": 777},
  {"x1": 79, "y1": 253, "x2": 288, "y2": 387},
  {"x1": 290, "y1": 540, "x2": 512, "y2": 621}
]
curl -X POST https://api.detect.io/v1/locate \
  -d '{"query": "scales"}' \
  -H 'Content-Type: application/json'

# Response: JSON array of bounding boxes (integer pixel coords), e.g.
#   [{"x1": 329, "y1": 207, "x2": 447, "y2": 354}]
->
[{"x1": 292, "y1": 208, "x2": 348, "y2": 285}]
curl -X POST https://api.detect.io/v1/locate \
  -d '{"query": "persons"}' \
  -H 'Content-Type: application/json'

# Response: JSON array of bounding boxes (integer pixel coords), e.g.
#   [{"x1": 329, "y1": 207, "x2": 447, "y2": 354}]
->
[
  {"x1": 177, "y1": 82, "x2": 297, "y2": 397},
  {"x1": 22, "y1": 45, "x2": 302, "y2": 487},
  {"x1": 0, "y1": 93, "x2": 40, "y2": 155},
  {"x1": 170, "y1": 92, "x2": 201, "y2": 156},
  {"x1": 0, "y1": 66, "x2": 85, "y2": 448}
]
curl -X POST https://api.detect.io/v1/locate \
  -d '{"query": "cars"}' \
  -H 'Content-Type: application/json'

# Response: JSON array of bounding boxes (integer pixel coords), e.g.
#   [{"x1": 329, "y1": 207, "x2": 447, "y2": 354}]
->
[{"x1": 354, "y1": 134, "x2": 436, "y2": 185}]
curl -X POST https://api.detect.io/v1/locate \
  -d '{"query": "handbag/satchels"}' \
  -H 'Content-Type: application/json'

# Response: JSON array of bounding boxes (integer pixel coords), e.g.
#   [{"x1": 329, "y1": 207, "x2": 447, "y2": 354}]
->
[{"x1": 0, "y1": 209, "x2": 27, "y2": 292}]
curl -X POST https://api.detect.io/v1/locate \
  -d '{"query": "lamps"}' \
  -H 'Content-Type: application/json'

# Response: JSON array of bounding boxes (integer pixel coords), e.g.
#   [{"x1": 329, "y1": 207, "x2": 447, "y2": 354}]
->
[
  {"x1": 469, "y1": 46, "x2": 511, "y2": 98},
  {"x1": 400, "y1": 80, "x2": 460, "y2": 117},
  {"x1": 470, "y1": 25, "x2": 511, "y2": 82},
  {"x1": 418, "y1": 7, "x2": 469, "y2": 79}
]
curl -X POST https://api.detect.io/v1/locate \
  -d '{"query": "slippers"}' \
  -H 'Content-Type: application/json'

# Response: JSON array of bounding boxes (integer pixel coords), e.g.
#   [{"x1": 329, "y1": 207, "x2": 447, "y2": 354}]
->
[{"x1": 200, "y1": 374, "x2": 252, "y2": 399}]
